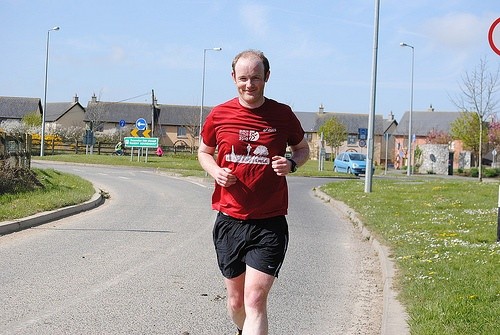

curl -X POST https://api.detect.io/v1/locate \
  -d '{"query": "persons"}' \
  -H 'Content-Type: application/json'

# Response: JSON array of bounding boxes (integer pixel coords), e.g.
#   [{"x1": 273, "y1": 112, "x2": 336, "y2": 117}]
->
[
  {"x1": 156, "y1": 146, "x2": 163, "y2": 157},
  {"x1": 198, "y1": 51, "x2": 309, "y2": 335},
  {"x1": 115, "y1": 141, "x2": 126, "y2": 155}
]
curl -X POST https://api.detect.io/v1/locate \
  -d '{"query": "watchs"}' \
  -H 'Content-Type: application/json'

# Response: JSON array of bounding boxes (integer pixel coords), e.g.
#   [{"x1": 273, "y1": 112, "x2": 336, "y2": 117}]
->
[{"x1": 289, "y1": 159, "x2": 297, "y2": 173}]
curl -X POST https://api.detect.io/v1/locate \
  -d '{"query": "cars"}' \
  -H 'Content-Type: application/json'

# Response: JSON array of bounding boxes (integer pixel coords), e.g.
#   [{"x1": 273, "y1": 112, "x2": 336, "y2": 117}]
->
[{"x1": 333, "y1": 152, "x2": 376, "y2": 176}]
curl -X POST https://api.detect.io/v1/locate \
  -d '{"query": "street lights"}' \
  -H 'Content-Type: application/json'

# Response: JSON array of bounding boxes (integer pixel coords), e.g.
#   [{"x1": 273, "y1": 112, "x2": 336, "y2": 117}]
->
[
  {"x1": 199, "y1": 48, "x2": 222, "y2": 146},
  {"x1": 399, "y1": 42, "x2": 414, "y2": 176},
  {"x1": 40, "y1": 27, "x2": 60, "y2": 156}
]
